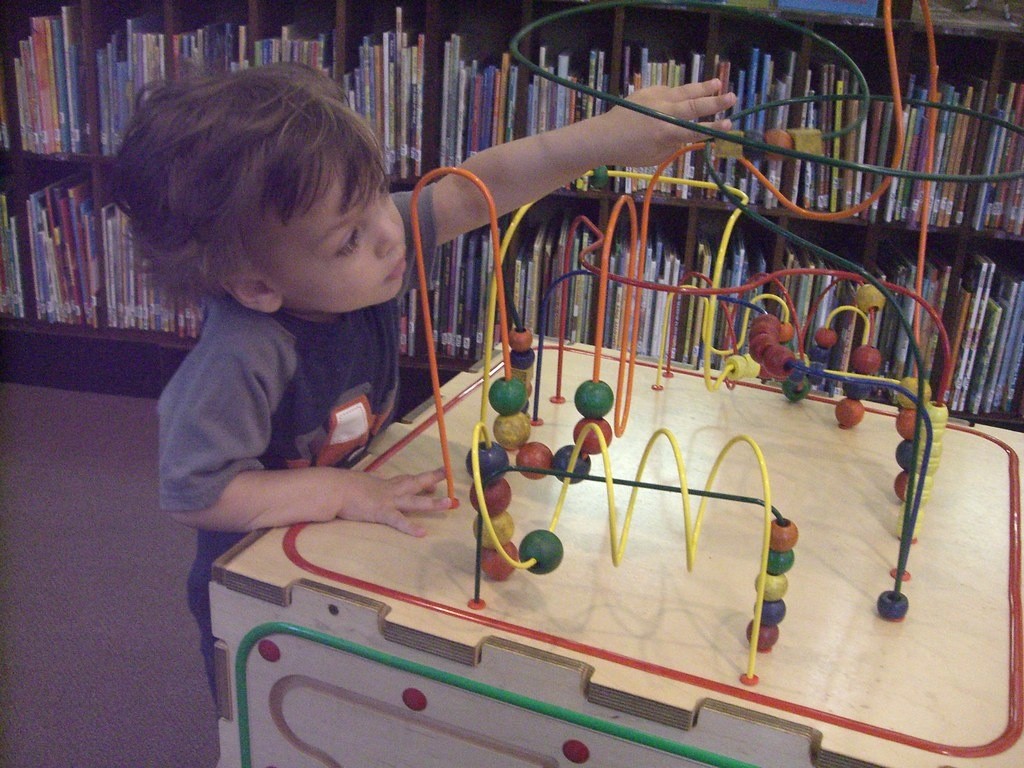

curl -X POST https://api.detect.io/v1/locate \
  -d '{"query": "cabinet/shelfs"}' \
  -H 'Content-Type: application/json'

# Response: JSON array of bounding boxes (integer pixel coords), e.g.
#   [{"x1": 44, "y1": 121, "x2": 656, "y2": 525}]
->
[{"x1": 0, "y1": 1, "x2": 1024, "y2": 427}]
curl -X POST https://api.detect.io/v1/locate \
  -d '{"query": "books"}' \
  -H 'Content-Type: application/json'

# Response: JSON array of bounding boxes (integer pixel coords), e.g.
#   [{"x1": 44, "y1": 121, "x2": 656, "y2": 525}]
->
[{"x1": 0, "y1": 1, "x2": 1024, "y2": 418}]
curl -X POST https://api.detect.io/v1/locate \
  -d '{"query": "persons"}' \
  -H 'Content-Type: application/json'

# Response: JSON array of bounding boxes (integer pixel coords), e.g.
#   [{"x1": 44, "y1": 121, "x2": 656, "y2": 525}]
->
[{"x1": 111, "y1": 61, "x2": 735, "y2": 712}]
